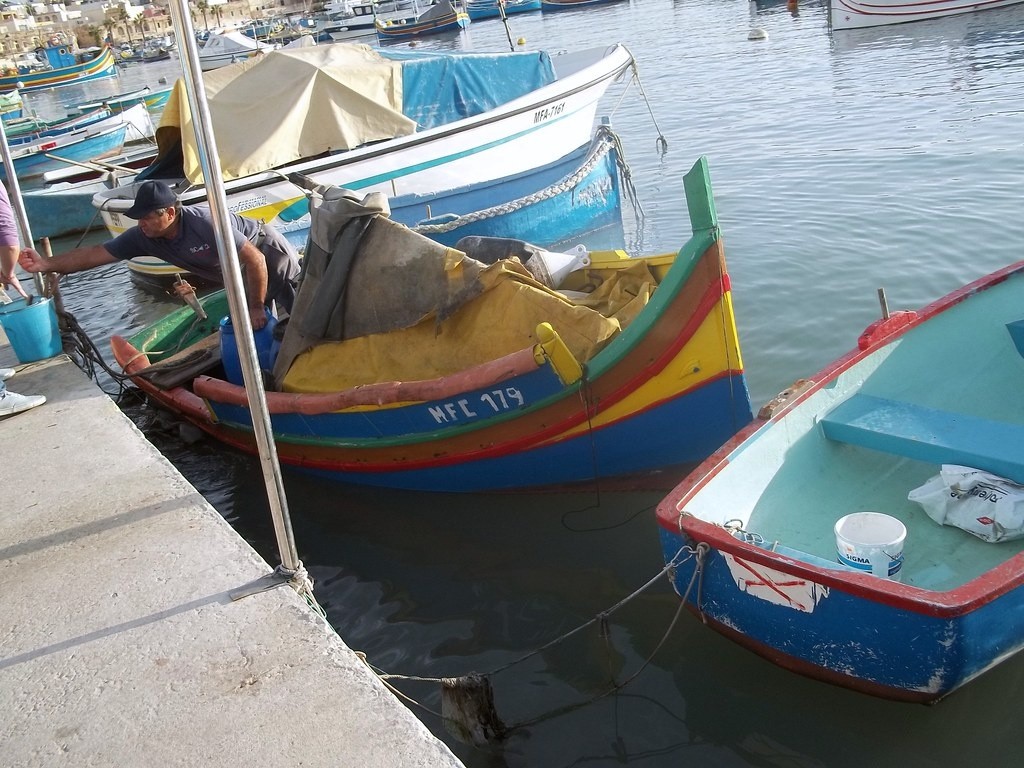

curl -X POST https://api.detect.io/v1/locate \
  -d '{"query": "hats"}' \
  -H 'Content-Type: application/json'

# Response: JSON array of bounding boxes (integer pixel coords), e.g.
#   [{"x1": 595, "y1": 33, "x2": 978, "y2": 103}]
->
[{"x1": 122, "y1": 182, "x2": 179, "y2": 218}]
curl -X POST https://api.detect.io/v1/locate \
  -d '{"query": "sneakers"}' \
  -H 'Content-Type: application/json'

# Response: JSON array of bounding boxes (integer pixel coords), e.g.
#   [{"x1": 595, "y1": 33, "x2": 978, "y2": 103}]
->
[
  {"x1": 0, "y1": 369, "x2": 15, "y2": 380},
  {"x1": 0, "y1": 391, "x2": 46, "y2": 416}
]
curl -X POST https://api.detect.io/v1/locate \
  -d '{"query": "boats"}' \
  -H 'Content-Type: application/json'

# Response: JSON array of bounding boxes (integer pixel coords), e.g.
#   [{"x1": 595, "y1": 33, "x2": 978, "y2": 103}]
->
[
  {"x1": 1, "y1": 0, "x2": 637, "y2": 292},
  {"x1": 831, "y1": 1, "x2": 1022, "y2": 29},
  {"x1": 104, "y1": 155, "x2": 754, "y2": 495},
  {"x1": 654, "y1": 259, "x2": 1024, "y2": 704}
]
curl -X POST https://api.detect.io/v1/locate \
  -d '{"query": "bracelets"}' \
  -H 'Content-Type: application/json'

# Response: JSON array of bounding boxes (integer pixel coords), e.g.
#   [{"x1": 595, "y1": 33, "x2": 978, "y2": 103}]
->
[{"x1": 248, "y1": 301, "x2": 265, "y2": 309}]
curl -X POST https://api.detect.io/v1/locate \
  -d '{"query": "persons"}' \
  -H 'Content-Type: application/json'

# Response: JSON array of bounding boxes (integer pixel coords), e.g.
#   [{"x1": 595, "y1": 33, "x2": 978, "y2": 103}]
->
[
  {"x1": 0, "y1": 179, "x2": 46, "y2": 416},
  {"x1": 17, "y1": 179, "x2": 301, "y2": 332}
]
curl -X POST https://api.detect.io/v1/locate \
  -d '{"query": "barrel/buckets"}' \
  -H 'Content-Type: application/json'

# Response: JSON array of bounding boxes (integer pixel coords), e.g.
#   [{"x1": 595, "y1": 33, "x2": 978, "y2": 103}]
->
[
  {"x1": 220, "y1": 305, "x2": 278, "y2": 388},
  {"x1": 834, "y1": 512, "x2": 906, "y2": 583},
  {"x1": 0, "y1": 294, "x2": 62, "y2": 363}
]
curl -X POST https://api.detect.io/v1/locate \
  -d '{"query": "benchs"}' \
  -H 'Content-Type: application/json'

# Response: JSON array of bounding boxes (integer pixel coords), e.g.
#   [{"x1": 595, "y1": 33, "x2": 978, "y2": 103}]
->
[
  {"x1": 823, "y1": 393, "x2": 1024, "y2": 485},
  {"x1": 140, "y1": 331, "x2": 221, "y2": 391}
]
